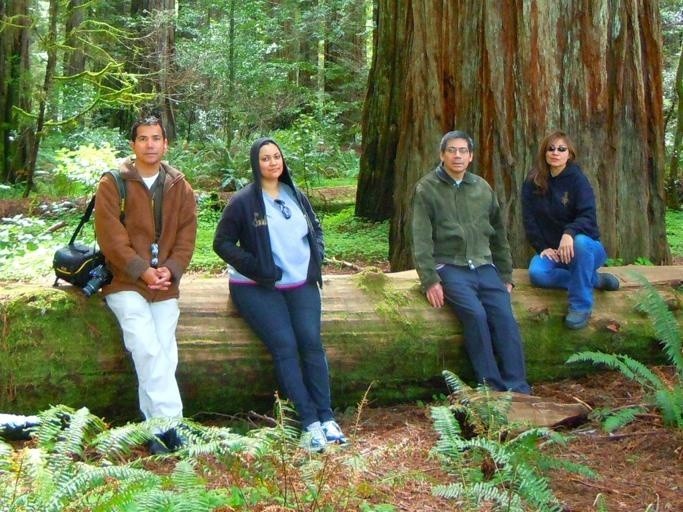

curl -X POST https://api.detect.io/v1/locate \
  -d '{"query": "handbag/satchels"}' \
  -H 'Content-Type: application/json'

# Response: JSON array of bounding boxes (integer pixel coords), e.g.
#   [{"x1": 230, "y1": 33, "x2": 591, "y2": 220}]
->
[{"x1": 52, "y1": 169, "x2": 127, "y2": 289}]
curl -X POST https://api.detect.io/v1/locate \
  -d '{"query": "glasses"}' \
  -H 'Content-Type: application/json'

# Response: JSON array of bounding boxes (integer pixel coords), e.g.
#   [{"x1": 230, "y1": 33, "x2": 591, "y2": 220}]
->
[
  {"x1": 444, "y1": 147, "x2": 469, "y2": 153},
  {"x1": 274, "y1": 198, "x2": 291, "y2": 220},
  {"x1": 150, "y1": 240, "x2": 159, "y2": 270},
  {"x1": 549, "y1": 145, "x2": 568, "y2": 151}
]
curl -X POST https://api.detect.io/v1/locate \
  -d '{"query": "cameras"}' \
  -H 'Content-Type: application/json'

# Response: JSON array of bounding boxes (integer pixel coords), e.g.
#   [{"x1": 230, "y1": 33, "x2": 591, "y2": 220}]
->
[{"x1": 82, "y1": 265, "x2": 113, "y2": 297}]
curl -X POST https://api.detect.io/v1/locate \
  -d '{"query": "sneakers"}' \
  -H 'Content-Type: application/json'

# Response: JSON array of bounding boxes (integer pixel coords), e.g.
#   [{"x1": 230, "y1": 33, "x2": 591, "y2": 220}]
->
[
  {"x1": 596, "y1": 273, "x2": 620, "y2": 292},
  {"x1": 304, "y1": 420, "x2": 328, "y2": 453},
  {"x1": 152, "y1": 423, "x2": 207, "y2": 461},
  {"x1": 321, "y1": 420, "x2": 347, "y2": 445},
  {"x1": 565, "y1": 310, "x2": 592, "y2": 330}
]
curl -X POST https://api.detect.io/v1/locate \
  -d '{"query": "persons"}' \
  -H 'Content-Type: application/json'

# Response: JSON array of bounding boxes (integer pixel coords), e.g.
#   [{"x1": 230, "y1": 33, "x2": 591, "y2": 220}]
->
[
  {"x1": 212, "y1": 138, "x2": 346, "y2": 457},
  {"x1": 412, "y1": 131, "x2": 530, "y2": 398},
  {"x1": 520, "y1": 133, "x2": 619, "y2": 329},
  {"x1": 94, "y1": 117, "x2": 198, "y2": 458}
]
